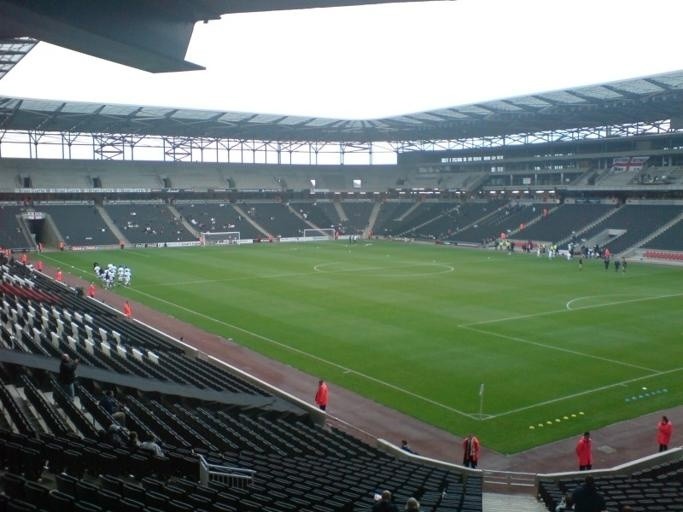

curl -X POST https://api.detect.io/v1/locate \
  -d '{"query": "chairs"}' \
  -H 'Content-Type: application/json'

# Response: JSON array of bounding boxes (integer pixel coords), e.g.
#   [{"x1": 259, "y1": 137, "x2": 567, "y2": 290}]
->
[
  {"x1": 538, "y1": 455, "x2": 683, "y2": 511},
  {"x1": 0, "y1": 263, "x2": 485, "y2": 511}
]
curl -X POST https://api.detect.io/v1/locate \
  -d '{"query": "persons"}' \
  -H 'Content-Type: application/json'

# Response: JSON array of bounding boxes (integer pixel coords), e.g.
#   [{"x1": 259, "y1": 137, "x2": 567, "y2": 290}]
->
[
  {"x1": 21, "y1": 255, "x2": 25, "y2": 265},
  {"x1": 54, "y1": 267, "x2": 63, "y2": 282},
  {"x1": 575, "y1": 431, "x2": 594, "y2": 470},
  {"x1": 92, "y1": 262, "x2": 131, "y2": 291},
  {"x1": 461, "y1": 433, "x2": 478, "y2": 470},
  {"x1": 654, "y1": 415, "x2": 671, "y2": 452},
  {"x1": 614, "y1": 260, "x2": 620, "y2": 272},
  {"x1": 622, "y1": 258, "x2": 627, "y2": 272},
  {"x1": 400, "y1": 439, "x2": 412, "y2": 453},
  {"x1": 403, "y1": 497, "x2": 420, "y2": 511},
  {"x1": 123, "y1": 300, "x2": 130, "y2": 316},
  {"x1": 314, "y1": 380, "x2": 328, "y2": 411},
  {"x1": 578, "y1": 256, "x2": 582, "y2": 270},
  {"x1": 97, "y1": 389, "x2": 125, "y2": 426},
  {"x1": 126, "y1": 432, "x2": 140, "y2": 447},
  {"x1": 88, "y1": 281, "x2": 96, "y2": 298},
  {"x1": 59, "y1": 241, "x2": 63, "y2": 251},
  {"x1": 494, "y1": 230, "x2": 610, "y2": 261},
  {"x1": 569, "y1": 475, "x2": 607, "y2": 511},
  {"x1": 59, "y1": 353, "x2": 80, "y2": 401},
  {"x1": 37, "y1": 261, "x2": 42, "y2": 272},
  {"x1": 372, "y1": 490, "x2": 400, "y2": 511},
  {"x1": 603, "y1": 258, "x2": 609, "y2": 272},
  {"x1": 140, "y1": 432, "x2": 166, "y2": 458}
]
